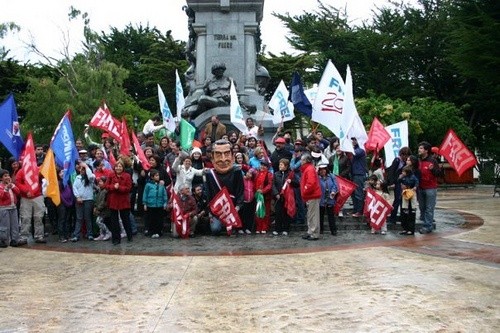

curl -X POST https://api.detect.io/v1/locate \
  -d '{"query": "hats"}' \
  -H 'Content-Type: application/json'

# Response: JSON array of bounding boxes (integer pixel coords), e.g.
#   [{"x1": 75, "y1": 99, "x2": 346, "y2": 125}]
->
[
  {"x1": 79, "y1": 150, "x2": 88, "y2": 154},
  {"x1": 275, "y1": 138, "x2": 285, "y2": 142},
  {"x1": 295, "y1": 140, "x2": 304, "y2": 146},
  {"x1": 191, "y1": 147, "x2": 202, "y2": 155},
  {"x1": 102, "y1": 133, "x2": 108, "y2": 138}
]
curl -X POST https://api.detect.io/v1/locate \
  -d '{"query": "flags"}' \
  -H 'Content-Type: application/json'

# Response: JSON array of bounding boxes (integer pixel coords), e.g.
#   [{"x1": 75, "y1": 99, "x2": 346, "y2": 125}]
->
[
  {"x1": 15, "y1": 133, "x2": 41, "y2": 196},
  {"x1": 157, "y1": 84, "x2": 177, "y2": 131},
  {"x1": 0, "y1": 95, "x2": 25, "y2": 162},
  {"x1": 268, "y1": 80, "x2": 296, "y2": 124},
  {"x1": 40, "y1": 149, "x2": 60, "y2": 207},
  {"x1": 230, "y1": 78, "x2": 248, "y2": 134},
  {"x1": 288, "y1": 72, "x2": 312, "y2": 116},
  {"x1": 50, "y1": 110, "x2": 81, "y2": 187},
  {"x1": 311, "y1": 59, "x2": 345, "y2": 139},
  {"x1": 168, "y1": 190, "x2": 191, "y2": 239},
  {"x1": 281, "y1": 171, "x2": 296, "y2": 217},
  {"x1": 384, "y1": 120, "x2": 408, "y2": 169},
  {"x1": 334, "y1": 175, "x2": 358, "y2": 213},
  {"x1": 209, "y1": 186, "x2": 242, "y2": 235},
  {"x1": 175, "y1": 69, "x2": 186, "y2": 121},
  {"x1": 339, "y1": 66, "x2": 369, "y2": 155},
  {"x1": 439, "y1": 129, "x2": 478, "y2": 175}
]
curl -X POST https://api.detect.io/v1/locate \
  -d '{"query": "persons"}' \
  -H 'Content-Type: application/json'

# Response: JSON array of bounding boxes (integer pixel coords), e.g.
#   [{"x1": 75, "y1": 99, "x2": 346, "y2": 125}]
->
[
  {"x1": 204, "y1": 115, "x2": 227, "y2": 142},
  {"x1": 300, "y1": 153, "x2": 322, "y2": 240},
  {"x1": 191, "y1": 63, "x2": 257, "y2": 118},
  {"x1": 0, "y1": 129, "x2": 440, "y2": 249},
  {"x1": 493, "y1": 161, "x2": 500, "y2": 197},
  {"x1": 242, "y1": 117, "x2": 264, "y2": 141}
]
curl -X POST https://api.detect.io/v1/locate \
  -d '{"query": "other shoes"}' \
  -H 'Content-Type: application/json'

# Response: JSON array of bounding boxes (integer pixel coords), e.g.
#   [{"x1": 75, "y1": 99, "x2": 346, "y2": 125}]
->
[
  {"x1": 58, "y1": 237, "x2": 67, "y2": 242},
  {"x1": 95, "y1": 220, "x2": 336, "y2": 245},
  {"x1": 352, "y1": 212, "x2": 361, "y2": 218},
  {"x1": 371, "y1": 230, "x2": 375, "y2": 234},
  {"x1": 52, "y1": 231, "x2": 58, "y2": 235},
  {"x1": 419, "y1": 228, "x2": 429, "y2": 234},
  {"x1": 0, "y1": 241, "x2": 8, "y2": 247},
  {"x1": 71, "y1": 236, "x2": 80, "y2": 242},
  {"x1": 10, "y1": 241, "x2": 23, "y2": 247},
  {"x1": 399, "y1": 231, "x2": 407, "y2": 235},
  {"x1": 66, "y1": 236, "x2": 70, "y2": 241},
  {"x1": 44, "y1": 233, "x2": 48, "y2": 236},
  {"x1": 35, "y1": 239, "x2": 47, "y2": 243},
  {"x1": 407, "y1": 232, "x2": 414, "y2": 235},
  {"x1": 380, "y1": 231, "x2": 385, "y2": 235},
  {"x1": 23, "y1": 239, "x2": 27, "y2": 244},
  {"x1": 346, "y1": 212, "x2": 353, "y2": 215},
  {"x1": 88, "y1": 236, "x2": 94, "y2": 240},
  {"x1": 338, "y1": 211, "x2": 343, "y2": 217}
]
